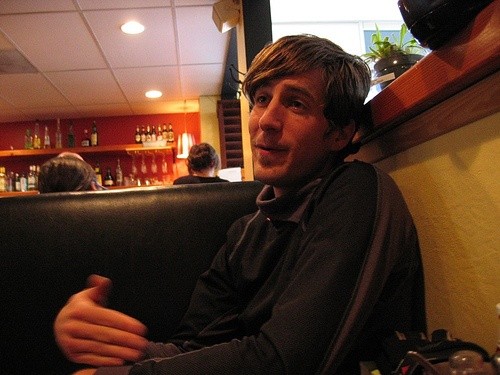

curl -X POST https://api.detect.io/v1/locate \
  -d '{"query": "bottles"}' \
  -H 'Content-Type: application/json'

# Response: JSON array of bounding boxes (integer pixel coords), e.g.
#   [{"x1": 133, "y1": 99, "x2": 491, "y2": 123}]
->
[
  {"x1": 90, "y1": 121, "x2": 98, "y2": 147},
  {"x1": 67, "y1": 125, "x2": 75, "y2": 148},
  {"x1": 80, "y1": 130, "x2": 91, "y2": 147},
  {"x1": 42, "y1": 126, "x2": 51, "y2": 149},
  {"x1": 447, "y1": 349, "x2": 496, "y2": 375},
  {"x1": 23, "y1": 128, "x2": 34, "y2": 150},
  {"x1": 490, "y1": 303, "x2": 500, "y2": 375},
  {"x1": 32, "y1": 119, "x2": 41, "y2": 150},
  {"x1": 135, "y1": 122, "x2": 174, "y2": 144},
  {"x1": 94, "y1": 161, "x2": 103, "y2": 186},
  {"x1": 115, "y1": 158, "x2": 123, "y2": 186},
  {"x1": 0, "y1": 164, "x2": 40, "y2": 192},
  {"x1": 104, "y1": 167, "x2": 115, "y2": 186},
  {"x1": 55, "y1": 118, "x2": 61, "y2": 148}
]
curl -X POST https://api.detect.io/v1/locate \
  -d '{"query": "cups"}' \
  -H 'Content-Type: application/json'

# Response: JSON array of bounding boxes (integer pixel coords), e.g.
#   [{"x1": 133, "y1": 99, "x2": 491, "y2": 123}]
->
[{"x1": 124, "y1": 175, "x2": 172, "y2": 186}]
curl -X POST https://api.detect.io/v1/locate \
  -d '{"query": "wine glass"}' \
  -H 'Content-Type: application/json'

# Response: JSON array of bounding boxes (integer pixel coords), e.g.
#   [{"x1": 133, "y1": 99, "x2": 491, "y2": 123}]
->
[{"x1": 131, "y1": 153, "x2": 168, "y2": 174}]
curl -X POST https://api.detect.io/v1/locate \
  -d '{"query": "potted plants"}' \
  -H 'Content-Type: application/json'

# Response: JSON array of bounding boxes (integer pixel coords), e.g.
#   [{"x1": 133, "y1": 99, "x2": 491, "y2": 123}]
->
[{"x1": 360, "y1": 24, "x2": 426, "y2": 91}]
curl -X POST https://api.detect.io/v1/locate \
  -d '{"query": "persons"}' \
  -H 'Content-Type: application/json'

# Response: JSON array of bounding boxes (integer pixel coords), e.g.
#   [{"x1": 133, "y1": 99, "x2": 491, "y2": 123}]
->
[
  {"x1": 173, "y1": 143, "x2": 229, "y2": 185},
  {"x1": 38, "y1": 155, "x2": 97, "y2": 194},
  {"x1": 54, "y1": 34, "x2": 428, "y2": 375}
]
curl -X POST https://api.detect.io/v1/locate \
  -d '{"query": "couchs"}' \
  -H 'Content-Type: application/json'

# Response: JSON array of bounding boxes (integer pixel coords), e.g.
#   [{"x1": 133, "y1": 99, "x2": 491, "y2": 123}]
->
[{"x1": 0, "y1": 180, "x2": 262, "y2": 375}]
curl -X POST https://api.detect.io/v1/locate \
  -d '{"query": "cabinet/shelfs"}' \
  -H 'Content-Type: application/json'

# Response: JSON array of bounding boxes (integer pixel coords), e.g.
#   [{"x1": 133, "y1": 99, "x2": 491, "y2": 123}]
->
[
  {"x1": 216, "y1": 99, "x2": 244, "y2": 170},
  {"x1": 0, "y1": 142, "x2": 178, "y2": 199}
]
